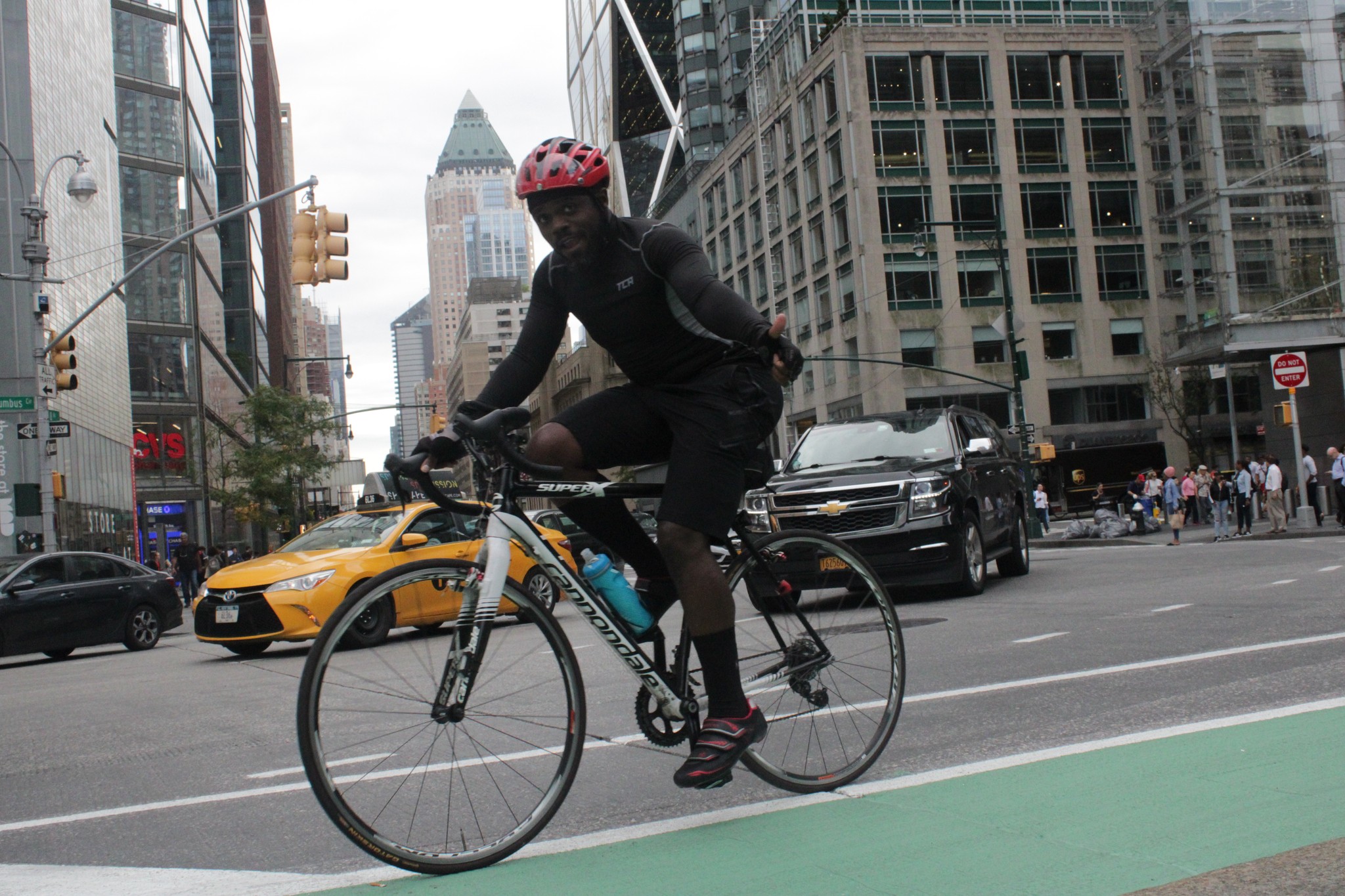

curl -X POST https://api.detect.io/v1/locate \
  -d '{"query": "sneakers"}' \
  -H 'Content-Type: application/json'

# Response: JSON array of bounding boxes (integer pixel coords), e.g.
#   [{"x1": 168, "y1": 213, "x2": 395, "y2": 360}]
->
[
  {"x1": 620, "y1": 572, "x2": 680, "y2": 639},
  {"x1": 672, "y1": 697, "x2": 768, "y2": 789}
]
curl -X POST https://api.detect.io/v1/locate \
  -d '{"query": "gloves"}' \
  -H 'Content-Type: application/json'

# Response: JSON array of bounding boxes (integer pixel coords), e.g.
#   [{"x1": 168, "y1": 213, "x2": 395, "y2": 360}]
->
[{"x1": 1133, "y1": 494, "x2": 1137, "y2": 499}]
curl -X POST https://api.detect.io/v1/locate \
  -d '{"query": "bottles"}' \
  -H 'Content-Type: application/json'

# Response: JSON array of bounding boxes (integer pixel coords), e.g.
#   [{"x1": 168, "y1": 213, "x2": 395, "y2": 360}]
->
[{"x1": 580, "y1": 548, "x2": 654, "y2": 635}]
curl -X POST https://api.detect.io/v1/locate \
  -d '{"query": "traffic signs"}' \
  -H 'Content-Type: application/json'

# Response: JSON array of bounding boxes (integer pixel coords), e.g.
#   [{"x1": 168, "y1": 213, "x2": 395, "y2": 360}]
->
[
  {"x1": 17, "y1": 421, "x2": 71, "y2": 440},
  {"x1": 44, "y1": 439, "x2": 58, "y2": 457},
  {"x1": 37, "y1": 363, "x2": 58, "y2": 399}
]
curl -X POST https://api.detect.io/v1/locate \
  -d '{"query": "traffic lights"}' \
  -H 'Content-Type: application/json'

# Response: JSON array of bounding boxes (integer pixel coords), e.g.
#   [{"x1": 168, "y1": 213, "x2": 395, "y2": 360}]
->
[
  {"x1": 316, "y1": 204, "x2": 349, "y2": 283},
  {"x1": 52, "y1": 473, "x2": 67, "y2": 499},
  {"x1": 429, "y1": 413, "x2": 446, "y2": 435},
  {"x1": 1035, "y1": 444, "x2": 1056, "y2": 461},
  {"x1": 1273, "y1": 404, "x2": 1292, "y2": 427},
  {"x1": 47, "y1": 331, "x2": 78, "y2": 395}
]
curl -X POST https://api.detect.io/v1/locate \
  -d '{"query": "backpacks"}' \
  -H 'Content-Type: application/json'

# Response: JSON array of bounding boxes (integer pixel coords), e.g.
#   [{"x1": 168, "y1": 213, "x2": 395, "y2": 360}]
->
[{"x1": 208, "y1": 554, "x2": 220, "y2": 571}]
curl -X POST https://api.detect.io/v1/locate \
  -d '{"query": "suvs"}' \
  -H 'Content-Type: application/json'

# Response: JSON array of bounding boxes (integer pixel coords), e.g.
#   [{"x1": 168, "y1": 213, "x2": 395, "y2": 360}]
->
[{"x1": 737, "y1": 405, "x2": 1031, "y2": 615}]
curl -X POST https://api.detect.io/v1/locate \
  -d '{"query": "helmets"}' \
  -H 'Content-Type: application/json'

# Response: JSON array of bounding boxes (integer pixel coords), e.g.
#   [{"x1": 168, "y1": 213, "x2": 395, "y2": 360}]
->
[{"x1": 513, "y1": 136, "x2": 613, "y2": 202}]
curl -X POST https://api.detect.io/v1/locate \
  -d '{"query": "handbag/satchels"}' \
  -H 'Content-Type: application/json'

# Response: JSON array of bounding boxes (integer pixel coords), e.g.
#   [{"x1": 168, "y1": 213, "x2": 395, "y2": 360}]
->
[
  {"x1": 1168, "y1": 509, "x2": 1185, "y2": 529},
  {"x1": 1132, "y1": 501, "x2": 1144, "y2": 511}
]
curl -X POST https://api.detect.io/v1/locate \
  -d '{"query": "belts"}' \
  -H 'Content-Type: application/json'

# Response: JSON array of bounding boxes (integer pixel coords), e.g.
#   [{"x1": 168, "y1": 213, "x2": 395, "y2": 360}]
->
[{"x1": 1266, "y1": 489, "x2": 1272, "y2": 491}]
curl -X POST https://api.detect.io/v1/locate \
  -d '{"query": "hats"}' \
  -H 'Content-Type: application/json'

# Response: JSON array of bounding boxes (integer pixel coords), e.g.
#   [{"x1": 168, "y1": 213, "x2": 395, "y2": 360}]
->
[
  {"x1": 1163, "y1": 466, "x2": 1177, "y2": 480},
  {"x1": 1214, "y1": 471, "x2": 1221, "y2": 476},
  {"x1": 1138, "y1": 474, "x2": 1145, "y2": 482},
  {"x1": 1198, "y1": 464, "x2": 1207, "y2": 470}
]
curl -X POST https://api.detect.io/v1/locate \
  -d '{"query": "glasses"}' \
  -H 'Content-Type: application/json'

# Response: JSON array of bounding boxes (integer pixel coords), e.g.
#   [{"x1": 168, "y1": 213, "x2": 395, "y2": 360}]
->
[{"x1": 1153, "y1": 475, "x2": 1157, "y2": 478}]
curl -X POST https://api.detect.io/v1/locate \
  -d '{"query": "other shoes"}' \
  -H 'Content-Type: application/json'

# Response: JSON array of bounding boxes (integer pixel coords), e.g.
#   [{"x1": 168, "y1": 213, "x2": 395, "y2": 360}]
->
[
  {"x1": 1337, "y1": 524, "x2": 1345, "y2": 529},
  {"x1": 1227, "y1": 513, "x2": 1232, "y2": 521},
  {"x1": 1320, "y1": 513, "x2": 1324, "y2": 521},
  {"x1": 1267, "y1": 529, "x2": 1286, "y2": 534},
  {"x1": 1232, "y1": 531, "x2": 1252, "y2": 538},
  {"x1": 1213, "y1": 534, "x2": 1231, "y2": 543}
]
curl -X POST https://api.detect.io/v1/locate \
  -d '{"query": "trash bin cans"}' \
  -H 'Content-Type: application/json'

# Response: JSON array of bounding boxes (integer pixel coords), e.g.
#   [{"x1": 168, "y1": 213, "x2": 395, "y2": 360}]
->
[
  {"x1": 1094, "y1": 496, "x2": 1120, "y2": 517},
  {"x1": 1137, "y1": 498, "x2": 1156, "y2": 516}
]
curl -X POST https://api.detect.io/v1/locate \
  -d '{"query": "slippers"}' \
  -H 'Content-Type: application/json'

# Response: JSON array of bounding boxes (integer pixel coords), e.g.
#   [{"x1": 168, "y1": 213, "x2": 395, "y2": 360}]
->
[{"x1": 1167, "y1": 543, "x2": 1180, "y2": 545}]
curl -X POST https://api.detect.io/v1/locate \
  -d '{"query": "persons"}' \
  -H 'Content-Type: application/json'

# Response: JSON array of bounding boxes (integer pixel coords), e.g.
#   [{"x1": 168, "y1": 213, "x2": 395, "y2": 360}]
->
[
  {"x1": 1326, "y1": 447, "x2": 1345, "y2": 530},
  {"x1": 197, "y1": 545, "x2": 254, "y2": 587},
  {"x1": 102, "y1": 547, "x2": 112, "y2": 554},
  {"x1": 1264, "y1": 455, "x2": 1287, "y2": 533},
  {"x1": 1210, "y1": 472, "x2": 1232, "y2": 543},
  {"x1": 1092, "y1": 482, "x2": 1105, "y2": 524},
  {"x1": 1144, "y1": 471, "x2": 1164, "y2": 510},
  {"x1": 1034, "y1": 484, "x2": 1051, "y2": 533},
  {"x1": 148, "y1": 551, "x2": 162, "y2": 571},
  {"x1": 172, "y1": 532, "x2": 202, "y2": 608},
  {"x1": 1223, "y1": 460, "x2": 1253, "y2": 538},
  {"x1": 408, "y1": 134, "x2": 804, "y2": 790},
  {"x1": 1127, "y1": 474, "x2": 1146, "y2": 521},
  {"x1": 1161, "y1": 466, "x2": 1182, "y2": 545},
  {"x1": 1172, "y1": 453, "x2": 1280, "y2": 527},
  {"x1": 1296, "y1": 444, "x2": 1324, "y2": 527}
]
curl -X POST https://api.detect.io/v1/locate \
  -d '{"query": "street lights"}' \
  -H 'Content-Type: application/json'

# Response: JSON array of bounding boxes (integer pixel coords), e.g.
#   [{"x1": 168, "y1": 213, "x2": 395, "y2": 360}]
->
[
  {"x1": 286, "y1": 355, "x2": 354, "y2": 541},
  {"x1": 911, "y1": 214, "x2": 1046, "y2": 539},
  {"x1": 0, "y1": 146, "x2": 99, "y2": 554},
  {"x1": 308, "y1": 411, "x2": 354, "y2": 521}
]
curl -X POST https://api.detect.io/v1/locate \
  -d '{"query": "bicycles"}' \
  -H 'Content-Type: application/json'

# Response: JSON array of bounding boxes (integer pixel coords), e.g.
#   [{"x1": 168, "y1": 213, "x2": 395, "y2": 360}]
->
[{"x1": 295, "y1": 398, "x2": 910, "y2": 875}]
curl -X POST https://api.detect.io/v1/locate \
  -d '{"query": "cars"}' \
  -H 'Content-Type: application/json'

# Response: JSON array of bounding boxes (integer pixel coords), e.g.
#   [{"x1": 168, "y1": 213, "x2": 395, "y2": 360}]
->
[
  {"x1": 0, "y1": 551, "x2": 185, "y2": 661},
  {"x1": 190, "y1": 468, "x2": 579, "y2": 659},
  {"x1": 522, "y1": 508, "x2": 624, "y2": 581},
  {"x1": 630, "y1": 511, "x2": 659, "y2": 545}
]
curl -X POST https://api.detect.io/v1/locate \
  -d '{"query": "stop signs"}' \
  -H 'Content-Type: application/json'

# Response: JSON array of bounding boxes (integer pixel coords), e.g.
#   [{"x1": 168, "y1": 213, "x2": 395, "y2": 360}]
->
[{"x1": 1273, "y1": 354, "x2": 1306, "y2": 387}]
